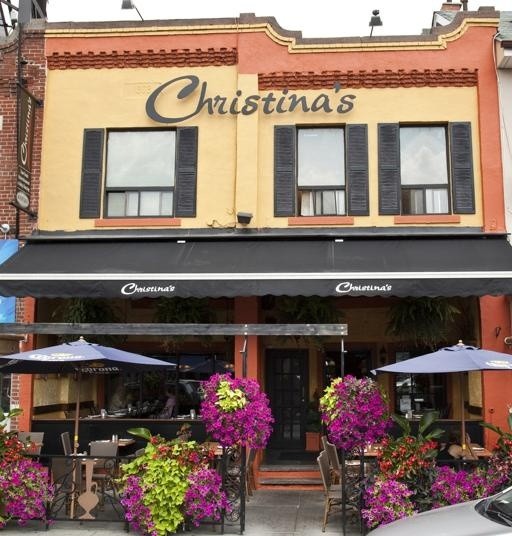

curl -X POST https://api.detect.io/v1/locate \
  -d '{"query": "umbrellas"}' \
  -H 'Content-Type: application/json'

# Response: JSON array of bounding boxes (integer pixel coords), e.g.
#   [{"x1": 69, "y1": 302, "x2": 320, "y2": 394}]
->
[
  {"x1": 0, "y1": 336, "x2": 178, "y2": 519},
  {"x1": 179, "y1": 359, "x2": 235, "y2": 380}
]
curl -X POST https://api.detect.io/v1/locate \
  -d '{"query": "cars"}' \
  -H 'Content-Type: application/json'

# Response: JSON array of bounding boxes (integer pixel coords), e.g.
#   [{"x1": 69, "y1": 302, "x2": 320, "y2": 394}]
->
[{"x1": 364, "y1": 486, "x2": 512, "y2": 535}]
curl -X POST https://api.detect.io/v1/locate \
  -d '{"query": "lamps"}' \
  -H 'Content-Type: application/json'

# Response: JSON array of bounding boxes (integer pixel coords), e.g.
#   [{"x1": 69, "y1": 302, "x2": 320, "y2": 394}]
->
[
  {"x1": 367, "y1": 9, "x2": 383, "y2": 36},
  {"x1": 121, "y1": 0, "x2": 143, "y2": 21},
  {"x1": 236, "y1": 212, "x2": 252, "y2": 224}
]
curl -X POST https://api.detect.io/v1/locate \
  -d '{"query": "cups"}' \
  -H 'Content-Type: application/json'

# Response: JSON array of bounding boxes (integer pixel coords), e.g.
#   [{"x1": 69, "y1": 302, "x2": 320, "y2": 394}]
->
[
  {"x1": 112, "y1": 434, "x2": 118, "y2": 441},
  {"x1": 406, "y1": 411, "x2": 412, "y2": 419},
  {"x1": 190, "y1": 410, "x2": 196, "y2": 418},
  {"x1": 101, "y1": 411, "x2": 107, "y2": 418}
]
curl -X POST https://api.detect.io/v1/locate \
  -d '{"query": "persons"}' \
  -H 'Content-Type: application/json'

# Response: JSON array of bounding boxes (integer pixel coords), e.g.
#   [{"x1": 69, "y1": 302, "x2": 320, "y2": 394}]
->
[
  {"x1": 434, "y1": 430, "x2": 479, "y2": 467},
  {"x1": 369, "y1": 340, "x2": 512, "y2": 459},
  {"x1": 146, "y1": 384, "x2": 178, "y2": 418},
  {"x1": 149, "y1": 386, "x2": 177, "y2": 418},
  {"x1": 109, "y1": 382, "x2": 130, "y2": 411}
]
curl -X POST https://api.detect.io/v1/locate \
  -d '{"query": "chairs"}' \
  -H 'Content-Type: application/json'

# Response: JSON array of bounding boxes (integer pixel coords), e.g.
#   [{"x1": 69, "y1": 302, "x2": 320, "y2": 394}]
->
[
  {"x1": 316, "y1": 436, "x2": 360, "y2": 533},
  {"x1": 59, "y1": 431, "x2": 114, "y2": 512}
]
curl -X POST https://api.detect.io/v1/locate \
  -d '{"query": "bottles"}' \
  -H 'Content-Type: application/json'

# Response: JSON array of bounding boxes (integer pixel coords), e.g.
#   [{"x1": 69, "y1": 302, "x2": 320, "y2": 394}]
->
[{"x1": 400, "y1": 395, "x2": 411, "y2": 412}]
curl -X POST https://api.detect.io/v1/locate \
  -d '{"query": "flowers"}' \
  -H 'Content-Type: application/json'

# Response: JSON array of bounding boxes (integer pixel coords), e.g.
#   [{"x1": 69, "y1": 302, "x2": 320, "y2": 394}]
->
[
  {"x1": 113, "y1": 424, "x2": 233, "y2": 536},
  {"x1": 197, "y1": 372, "x2": 275, "y2": 450},
  {"x1": 318, "y1": 373, "x2": 388, "y2": 454},
  {"x1": 0, "y1": 406, "x2": 61, "y2": 528},
  {"x1": 360, "y1": 411, "x2": 512, "y2": 532}
]
